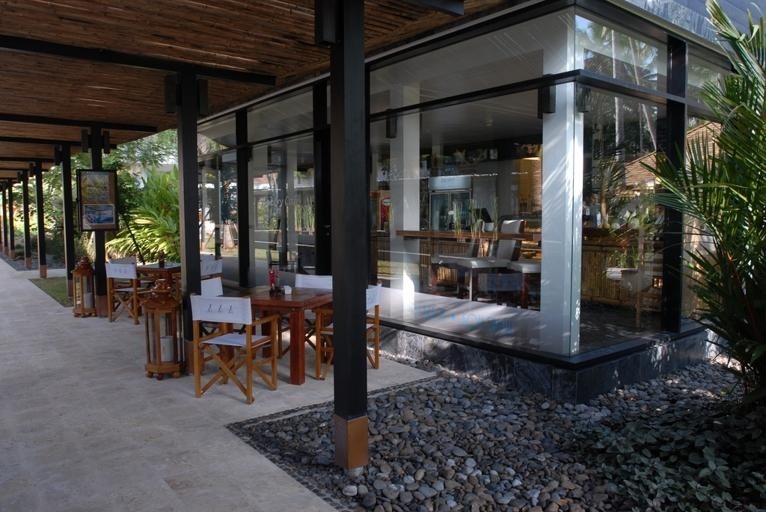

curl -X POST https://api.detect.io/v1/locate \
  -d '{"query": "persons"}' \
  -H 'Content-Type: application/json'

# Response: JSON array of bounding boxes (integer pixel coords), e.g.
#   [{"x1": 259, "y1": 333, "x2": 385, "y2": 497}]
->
[{"x1": 583, "y1": 191, "x2": 599, "y2": 212}]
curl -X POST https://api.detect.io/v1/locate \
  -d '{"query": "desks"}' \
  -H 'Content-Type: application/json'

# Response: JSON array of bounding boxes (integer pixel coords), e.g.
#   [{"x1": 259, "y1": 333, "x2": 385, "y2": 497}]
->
[
  {"x1": 137, "y1": 261, "x2": 181, "y2": 302},
  {"x1": 234, "y1": 285, "x2": 333, "y2": 385}
]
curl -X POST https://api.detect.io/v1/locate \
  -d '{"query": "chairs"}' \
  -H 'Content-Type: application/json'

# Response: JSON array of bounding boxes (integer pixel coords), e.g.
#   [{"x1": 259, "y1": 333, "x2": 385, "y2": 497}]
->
[
  {"x1": 103, "y1": 255, "x2": 155, "y2": 325},
  {"x1": 189, "y1": 294, "x2": 279, "y2": 404},
  {"x1": 200, "y1": 278, "x2": 258, "y2": 367},
  {"x1": 430, "y1": 218, "x2": 525, "y2": 303},
  {"x1": 311, "y1": 283, "x2": 381, "y2": 383}
]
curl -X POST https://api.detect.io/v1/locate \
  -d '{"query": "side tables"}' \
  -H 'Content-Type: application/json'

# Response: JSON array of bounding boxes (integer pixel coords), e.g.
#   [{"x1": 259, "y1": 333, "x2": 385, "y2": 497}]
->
[{"x1": 506, "y1": 260, "x2": 541, "y2": 309}]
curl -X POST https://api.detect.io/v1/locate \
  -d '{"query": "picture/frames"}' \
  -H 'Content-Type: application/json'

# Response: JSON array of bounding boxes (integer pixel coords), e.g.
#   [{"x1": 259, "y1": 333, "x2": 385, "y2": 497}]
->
[{"x1": 77, "y1": 169, "x2": 118, "y2": 232}]
